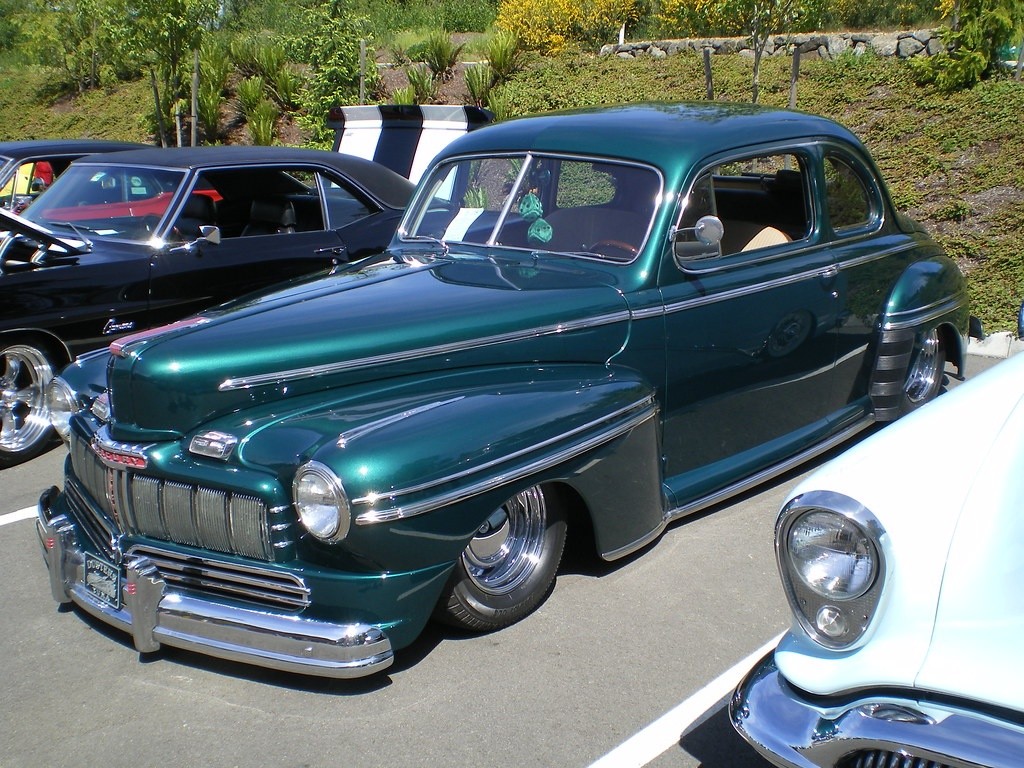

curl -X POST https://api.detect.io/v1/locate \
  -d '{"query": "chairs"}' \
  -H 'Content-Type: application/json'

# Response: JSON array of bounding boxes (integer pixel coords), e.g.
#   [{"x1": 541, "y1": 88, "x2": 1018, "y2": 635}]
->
[
  {"x1": 174, "y1": 194, "x2": 217, "y2": 241},
  {"x1": 240, "y1": 194, "x2": 297, "y2": 237}
]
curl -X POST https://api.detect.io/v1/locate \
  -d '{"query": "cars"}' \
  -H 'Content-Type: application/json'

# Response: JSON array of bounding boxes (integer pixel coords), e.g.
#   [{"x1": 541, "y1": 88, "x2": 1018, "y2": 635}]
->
[
  {"x1": 728, "y1": 292, "x2": 1024, "y2": 768},
  {"x1": 0, "y1": 145, "x2": 525, "y2": 466},
  {"x1": 0, "y1": 139, "x2": 221, "y2": 224},
  {"x1": 36, "y1": 100, "x2": 984, "y2": 681}
]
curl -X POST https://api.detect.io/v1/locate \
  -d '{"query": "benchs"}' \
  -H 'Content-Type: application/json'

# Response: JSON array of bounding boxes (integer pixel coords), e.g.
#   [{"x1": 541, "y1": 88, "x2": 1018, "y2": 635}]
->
[{"x1": 530, "y1": 207, "x2": 648, "y2": 258}]
